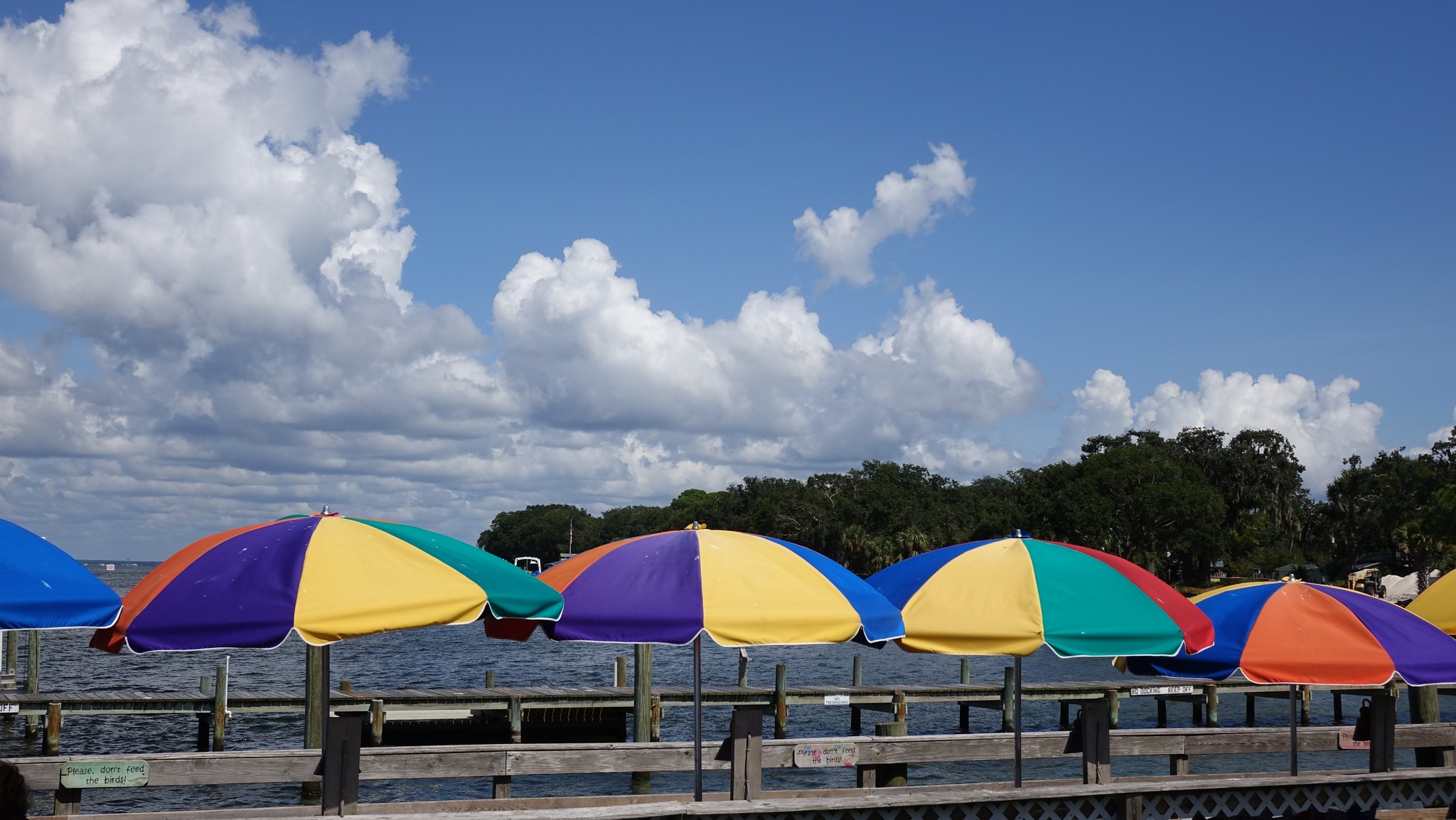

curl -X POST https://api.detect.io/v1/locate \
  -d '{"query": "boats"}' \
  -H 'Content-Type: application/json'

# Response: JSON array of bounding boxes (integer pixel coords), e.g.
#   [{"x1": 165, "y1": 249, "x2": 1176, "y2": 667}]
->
[{"x1": 514, "y1": 556, "x2": 541, "y2": 577}]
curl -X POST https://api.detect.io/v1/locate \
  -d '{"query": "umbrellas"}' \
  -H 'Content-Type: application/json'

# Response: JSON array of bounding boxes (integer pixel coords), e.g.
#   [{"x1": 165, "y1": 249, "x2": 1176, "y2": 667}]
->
[
  {"x1": 1403, "y1": 563, "x2": 1456, "y2": 635},
  {"x1": 857, "y1": 523, "x2": 1220, "y2": 787},
  {"x1": 479, "y1": 521, "x2": 906, "y2": 803},
  {"x1": 0, "y1": 518, "x2": 125, "y2": 639},
  {"x1": 1113, "y1": 569, "x2": 1456, "y2": 779},
  {"x1": 91, "y1": 499, "x2": 565, "y2": 755}
]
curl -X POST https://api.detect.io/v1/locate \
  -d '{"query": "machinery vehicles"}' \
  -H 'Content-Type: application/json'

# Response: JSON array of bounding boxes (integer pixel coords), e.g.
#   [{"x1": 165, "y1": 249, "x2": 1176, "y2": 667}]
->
[{"x1": 1345, "y1": 568, "x2": 1387, "y2": 599}]
